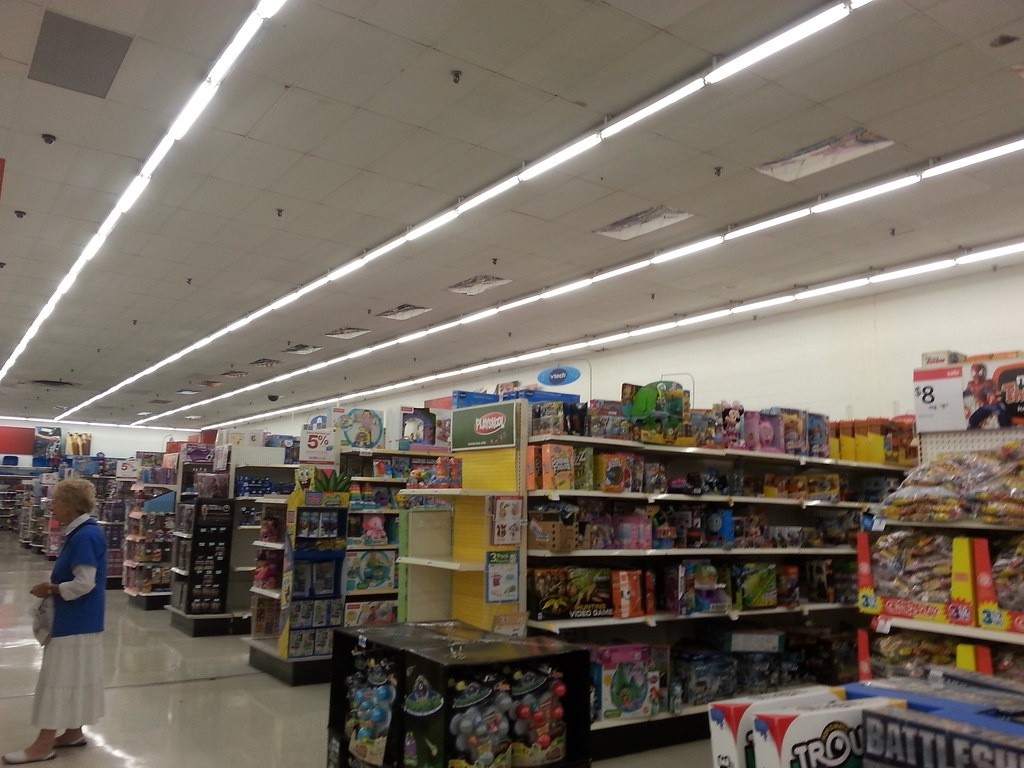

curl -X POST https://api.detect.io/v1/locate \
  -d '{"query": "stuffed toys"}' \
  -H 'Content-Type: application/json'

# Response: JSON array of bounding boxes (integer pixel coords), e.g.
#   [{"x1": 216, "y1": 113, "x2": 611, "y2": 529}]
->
[{"x1": 364, "y1": 514, "x2": 386, "y2": 539}]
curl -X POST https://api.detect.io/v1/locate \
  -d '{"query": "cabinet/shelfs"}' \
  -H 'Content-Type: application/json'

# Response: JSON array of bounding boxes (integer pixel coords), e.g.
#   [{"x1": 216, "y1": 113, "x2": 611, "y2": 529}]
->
[{"x1": 0, "y1": 382, "x2": 1024, "y2": 768}]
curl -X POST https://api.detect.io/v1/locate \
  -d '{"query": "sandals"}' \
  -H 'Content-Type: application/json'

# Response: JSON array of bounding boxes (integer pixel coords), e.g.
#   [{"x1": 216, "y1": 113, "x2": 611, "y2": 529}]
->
[
  {"x1": 52, "y1": 735, "x2": 89, "y2": 748},
  {"x1": 4, "y1": 748, "x2": 56, "y2": 764}
]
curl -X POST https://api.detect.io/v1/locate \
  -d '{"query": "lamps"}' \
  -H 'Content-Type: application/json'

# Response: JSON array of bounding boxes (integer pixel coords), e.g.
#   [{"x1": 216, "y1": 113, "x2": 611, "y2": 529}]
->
[{"x1": 1, "y1": 2, "x2": 1024, "y2": 435}]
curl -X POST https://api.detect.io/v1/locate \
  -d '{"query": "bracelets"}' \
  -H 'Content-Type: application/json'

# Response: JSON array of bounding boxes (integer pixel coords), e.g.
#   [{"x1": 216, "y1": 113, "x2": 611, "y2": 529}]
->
[{"x1": 47, "y1": 582, "x2": 53, "y2": 594}]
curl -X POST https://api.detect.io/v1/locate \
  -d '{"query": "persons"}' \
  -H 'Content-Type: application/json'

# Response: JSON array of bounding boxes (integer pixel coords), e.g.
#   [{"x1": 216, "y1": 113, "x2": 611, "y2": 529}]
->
[
  {"x1": 252, "y1": 517, "x2": 280, "y2": 592},
  {"x1": 66, "y1": 432, "x2": 91, "y2": 456},
  {"x1": 2, "y1": 477, "x2": 108, "y2": 765},
  {"x1": 33, "y1": 428, "x2": 60, "y2": 455}
]
